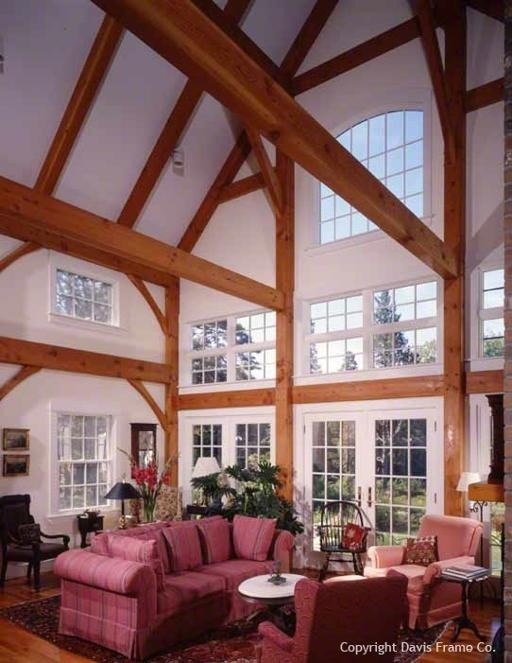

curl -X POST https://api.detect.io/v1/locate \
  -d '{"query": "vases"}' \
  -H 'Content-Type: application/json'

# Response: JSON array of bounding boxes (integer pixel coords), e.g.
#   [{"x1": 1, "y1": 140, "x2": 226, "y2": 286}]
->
[{"x1": 141, "y1": 489, "x2": 158, "y2": 521}]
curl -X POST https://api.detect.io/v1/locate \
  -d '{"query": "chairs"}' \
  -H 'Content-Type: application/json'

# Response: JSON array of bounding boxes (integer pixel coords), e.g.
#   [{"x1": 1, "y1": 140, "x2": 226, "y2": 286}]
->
[
  {"x1": 315, "y1": 499, "x2": 371, "y2": 579},
  {"x1": 0, "y1": 494, "x2": 72, "y2": 586}
]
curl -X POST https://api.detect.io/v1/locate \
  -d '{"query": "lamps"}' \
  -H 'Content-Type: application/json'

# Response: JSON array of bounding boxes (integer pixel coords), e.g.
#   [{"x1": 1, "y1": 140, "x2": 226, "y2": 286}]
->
[
  {"x1": 103, "y1": 482, "x2": 142, "y2": 527},
  {"x1": 192, "y1": 455, "x2": 221, "y2": 501},
  {"x1": 454, "y1": 471, "x2": 482, "y2": 518}
]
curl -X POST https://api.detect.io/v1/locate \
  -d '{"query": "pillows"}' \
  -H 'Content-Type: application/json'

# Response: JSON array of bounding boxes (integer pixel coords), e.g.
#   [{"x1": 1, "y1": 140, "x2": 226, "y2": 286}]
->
[
  {"x1": 19, "y1": 522, "x2": 43, "y2": 545},
  {"x1": 89, "y1": 513, "x2": 278, "y2": 583},
  {"x1": 401, "y1": 535, "x2": 438, "y2": 565}
]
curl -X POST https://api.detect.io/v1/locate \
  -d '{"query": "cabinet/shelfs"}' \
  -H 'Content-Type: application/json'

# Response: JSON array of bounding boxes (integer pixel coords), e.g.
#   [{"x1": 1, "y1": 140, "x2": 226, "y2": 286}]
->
[
  {"x1": 78, "y1": 515, "x2": 104, "y2": 548},
  {"x1": 129, "y1": 421, "x2": 158, "y2": 478}
]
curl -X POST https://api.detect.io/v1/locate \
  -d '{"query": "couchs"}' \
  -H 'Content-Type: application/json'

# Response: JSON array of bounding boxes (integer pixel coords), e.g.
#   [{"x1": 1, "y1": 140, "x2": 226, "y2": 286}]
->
[
  {"x1": 53, "y1": 513, "x2": 297, "y2": 659},
  {"x1": 258, "y1": 568, "x2": 409, "y2": 663},
  {"x1": 364, "y1": 514, "x2": 485, "y2": 633}
]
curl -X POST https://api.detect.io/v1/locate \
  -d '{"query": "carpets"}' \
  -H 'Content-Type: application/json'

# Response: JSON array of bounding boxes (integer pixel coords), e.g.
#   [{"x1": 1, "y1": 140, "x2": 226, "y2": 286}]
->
[{"x1": 1, "y1": 576, "x2": 451, "y2": 663}]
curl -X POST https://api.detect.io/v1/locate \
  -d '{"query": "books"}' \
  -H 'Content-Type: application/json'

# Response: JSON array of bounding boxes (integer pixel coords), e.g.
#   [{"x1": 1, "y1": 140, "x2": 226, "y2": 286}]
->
[{"x1": 441, "y1": 563, "x2": 491, "y2": 582}]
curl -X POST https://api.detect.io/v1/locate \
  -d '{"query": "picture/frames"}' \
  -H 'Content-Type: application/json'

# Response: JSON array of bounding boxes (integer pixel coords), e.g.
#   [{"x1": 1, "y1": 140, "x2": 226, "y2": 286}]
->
[
  {"x1": 3, "y1": 426, "x2": 31, "y2": 449},
  {"x1": 3, "y1": 454, "x2": 31, "y2": 478}
]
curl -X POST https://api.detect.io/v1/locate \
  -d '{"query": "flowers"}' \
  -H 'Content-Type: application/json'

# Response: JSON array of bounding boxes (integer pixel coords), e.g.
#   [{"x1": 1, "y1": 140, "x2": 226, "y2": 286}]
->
[{"x1": 116, "y1": 443, "x2": 183, "y2": 522}]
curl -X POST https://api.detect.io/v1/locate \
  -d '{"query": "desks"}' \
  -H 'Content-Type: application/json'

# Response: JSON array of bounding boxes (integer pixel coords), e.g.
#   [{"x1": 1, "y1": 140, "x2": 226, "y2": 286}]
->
[
  {"x1": 435, "y1": 573, "x2": 490, "y2": 645},
  {"x1": 188, "y1": 505, "x2": 235, "y2": 518}
]
